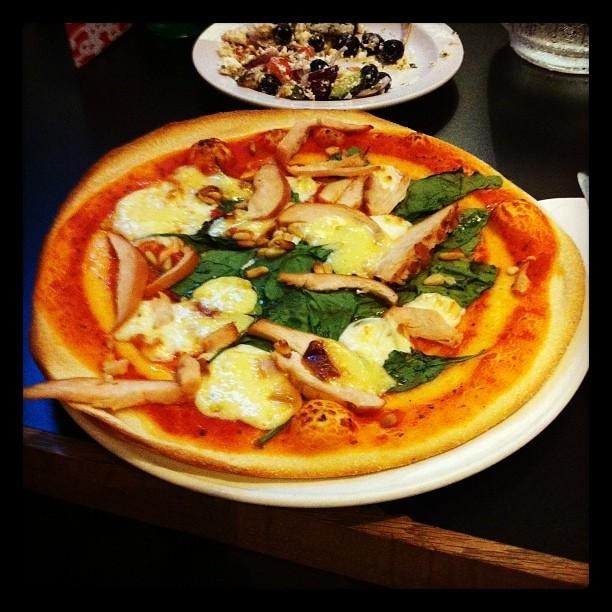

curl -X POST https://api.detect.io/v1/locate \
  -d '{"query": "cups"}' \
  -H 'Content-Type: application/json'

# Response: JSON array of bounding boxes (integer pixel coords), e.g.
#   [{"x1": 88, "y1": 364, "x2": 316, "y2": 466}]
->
[{"x1": 502, "y1": 23, "x2": 590, "y2": 76}]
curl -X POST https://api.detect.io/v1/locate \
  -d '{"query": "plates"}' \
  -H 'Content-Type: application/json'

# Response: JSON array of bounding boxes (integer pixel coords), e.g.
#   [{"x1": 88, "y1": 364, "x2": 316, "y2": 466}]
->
[
  {"x1": 41, "y1": 197, "x2": 590, "y2": 511},
  {"x1": 192, "y1": 23, "x2": 466, "y2": 112}
]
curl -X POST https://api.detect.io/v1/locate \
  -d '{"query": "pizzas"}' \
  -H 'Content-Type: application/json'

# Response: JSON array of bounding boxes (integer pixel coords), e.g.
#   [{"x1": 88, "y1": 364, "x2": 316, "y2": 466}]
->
[{"x1": 22, "y1": 107, "x2": 586, "y2": 479}]
